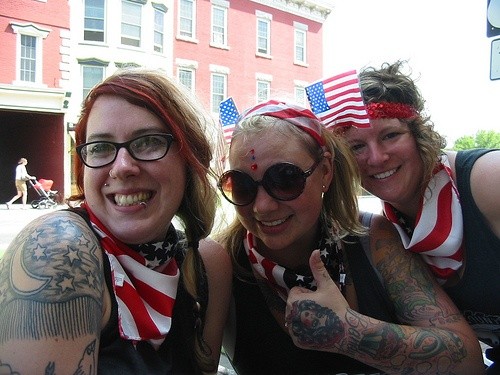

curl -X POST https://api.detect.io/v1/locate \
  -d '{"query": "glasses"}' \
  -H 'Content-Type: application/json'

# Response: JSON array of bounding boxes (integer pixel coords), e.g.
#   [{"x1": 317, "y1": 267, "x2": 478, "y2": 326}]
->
[
  {"x1": 217, "y1": 155, "x2": 325, "y2": 206},
  {"x1": 76, "y1": 134, "x2": 176, "y2": 168}
]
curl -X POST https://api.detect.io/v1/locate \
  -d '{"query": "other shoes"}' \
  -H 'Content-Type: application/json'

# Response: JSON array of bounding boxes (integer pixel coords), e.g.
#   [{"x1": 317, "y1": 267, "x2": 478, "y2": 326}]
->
[{"x1": 6, "y1": 202, "x2": 11, "y2": 210}]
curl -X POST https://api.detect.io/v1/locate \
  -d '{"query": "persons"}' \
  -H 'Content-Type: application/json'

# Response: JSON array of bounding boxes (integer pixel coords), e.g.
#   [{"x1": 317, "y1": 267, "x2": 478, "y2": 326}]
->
[
  {"x1": 329, "y1": 59, "x2": 500, "y2": 375},
  {"x1": 0, "y1": 71, "x2": 234, "y2": 375},
  {"x1": 6, "y1": 158, "x2": 37, "y2": 209},
  {"x1": 208, "y1": 100, "x2": 485, "y2": 375}
]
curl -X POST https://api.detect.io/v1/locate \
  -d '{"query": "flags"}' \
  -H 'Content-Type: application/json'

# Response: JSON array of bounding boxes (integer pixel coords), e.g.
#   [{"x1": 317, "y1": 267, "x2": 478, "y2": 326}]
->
[
  {"x1": 214, "y1": 97, "x2": 240, "y2": 191},
  {"x1": 304, "y1": 69, "x2": 373, "y2": 130}
]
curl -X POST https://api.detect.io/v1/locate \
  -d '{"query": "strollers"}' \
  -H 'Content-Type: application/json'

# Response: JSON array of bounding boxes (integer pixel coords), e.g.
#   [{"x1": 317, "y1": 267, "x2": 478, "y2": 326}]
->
[{"x1": 28, "y1": 178, "x2": 59, "y2": 210}]
